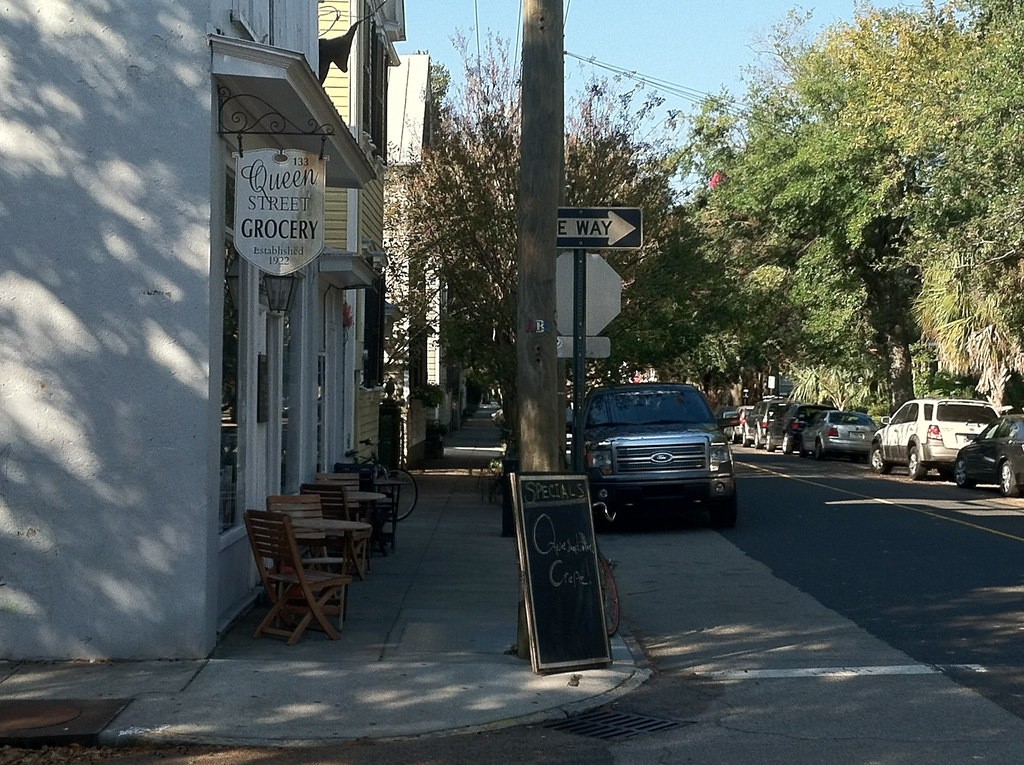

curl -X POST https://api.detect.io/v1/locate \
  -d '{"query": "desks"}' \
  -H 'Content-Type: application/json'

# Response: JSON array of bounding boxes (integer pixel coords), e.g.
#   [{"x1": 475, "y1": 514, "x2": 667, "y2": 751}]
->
[
  {"x1": 374, "y1": 479, "x2": 409, "y2": 554},
  {"x1": 290, "y1": 518, "x2": 373, "y2": 632},
  {"x1": 346, "y1": 491, "x2": 386, "y2": 576}
]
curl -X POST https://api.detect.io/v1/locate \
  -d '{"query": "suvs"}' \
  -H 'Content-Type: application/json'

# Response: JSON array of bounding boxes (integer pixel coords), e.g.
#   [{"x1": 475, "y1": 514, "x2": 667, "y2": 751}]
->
[
  {"x1": 765, "y1": 404, "x2": 836, "y2": 455},
  {"x1": 870, "y1": 398, "x2": 999, "y2": 481},
  {"x1": 583, "y1": 383, "x2": 737, "y2": 531}
]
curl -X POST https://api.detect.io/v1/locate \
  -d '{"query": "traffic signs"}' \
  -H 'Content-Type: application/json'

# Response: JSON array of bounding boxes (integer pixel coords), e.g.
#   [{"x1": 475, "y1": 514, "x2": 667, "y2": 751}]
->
[{"x1": 557, "y1": 206, "x2": 644, "y2": 249}]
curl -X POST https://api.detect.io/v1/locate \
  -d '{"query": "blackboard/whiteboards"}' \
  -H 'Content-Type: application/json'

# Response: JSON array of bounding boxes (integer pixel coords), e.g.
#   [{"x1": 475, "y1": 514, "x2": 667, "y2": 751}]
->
[{"x1": 507, "y1": 469, "x2": 615, "y2": 678}]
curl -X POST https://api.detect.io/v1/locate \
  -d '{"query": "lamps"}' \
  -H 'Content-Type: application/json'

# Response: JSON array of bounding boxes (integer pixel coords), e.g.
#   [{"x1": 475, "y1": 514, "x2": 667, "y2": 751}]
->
[{"x1": 263, "y1": 271, "x2": 302, "y2": 313}]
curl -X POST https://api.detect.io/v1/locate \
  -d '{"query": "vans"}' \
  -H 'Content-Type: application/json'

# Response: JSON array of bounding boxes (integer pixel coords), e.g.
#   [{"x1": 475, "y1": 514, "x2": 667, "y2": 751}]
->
[{"x1": 747, "y1": 401, "x2": 790, "y2": 448}]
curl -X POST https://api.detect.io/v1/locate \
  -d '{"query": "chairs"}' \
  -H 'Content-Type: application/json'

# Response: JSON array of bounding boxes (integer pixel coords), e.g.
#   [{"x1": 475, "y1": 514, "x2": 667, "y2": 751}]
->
[
  {"x1": 242, "y1": 508, "x2": 352, "y2": 646},
  {"x1": 313, "y1": 472, "x2": 360, "y2": 521},
  {"x1": 266, "y1": 494, "x2": 338, "y2": 606},
  {"x1": 334, "y1": 463, "x2": 388, "y2": 557},
  {"x1": 300, "y1": 483, "x2": 364, "y2": 582}
]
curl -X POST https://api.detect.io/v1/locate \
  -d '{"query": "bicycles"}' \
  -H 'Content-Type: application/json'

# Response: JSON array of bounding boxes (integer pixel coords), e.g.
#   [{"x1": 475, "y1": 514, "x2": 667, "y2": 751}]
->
[{"x1": 346, "y1": 438, "x2": 419, "y2": 523}]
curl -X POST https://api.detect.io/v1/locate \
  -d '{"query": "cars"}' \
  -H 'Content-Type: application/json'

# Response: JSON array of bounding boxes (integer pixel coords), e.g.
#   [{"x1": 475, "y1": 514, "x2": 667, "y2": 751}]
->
[
  {"x1": 953, "y1": 414, "x2": 1024, "y2": 498},
  {"x1": 800, "y1": 410, "x2": 878, "y2": 460},
  {"x1": 725, "y1": 405, "x2": 749, "y2": 444}
]
